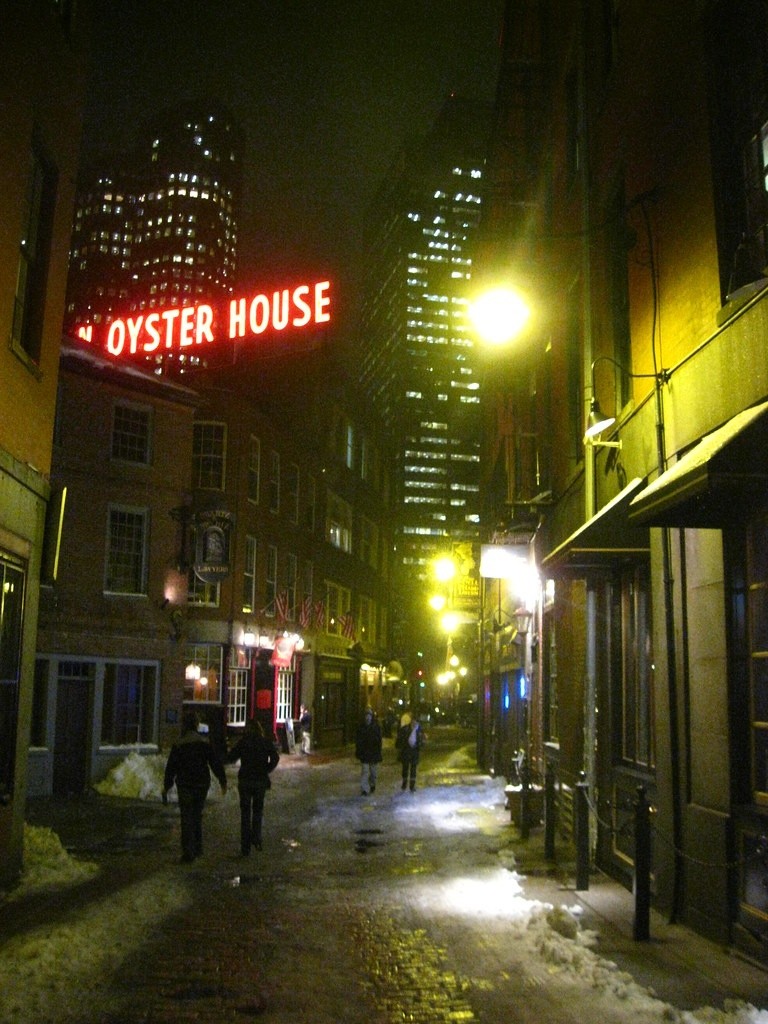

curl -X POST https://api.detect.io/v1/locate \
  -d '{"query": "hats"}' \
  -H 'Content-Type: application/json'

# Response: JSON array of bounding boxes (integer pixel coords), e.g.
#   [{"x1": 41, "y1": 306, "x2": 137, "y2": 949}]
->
[{"x1": 365, "y1": 708, "x2": 373, "y2": 715}]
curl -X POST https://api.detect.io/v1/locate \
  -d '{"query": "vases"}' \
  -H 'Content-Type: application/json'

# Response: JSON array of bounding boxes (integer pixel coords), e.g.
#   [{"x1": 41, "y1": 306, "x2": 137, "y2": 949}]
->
[{"x1": 503, "y1": 790, "x2": 545, "y2": 828}]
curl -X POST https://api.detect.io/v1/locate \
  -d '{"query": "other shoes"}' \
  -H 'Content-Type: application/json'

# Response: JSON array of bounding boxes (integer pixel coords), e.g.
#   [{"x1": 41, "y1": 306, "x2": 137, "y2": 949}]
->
[
  {"x1": 361, "y1": 791, "x2": 367, "y2": 797},
  {"x1": 410, "y1": 784, "x2": 416, "y2": 793},
  {"x1": 371, "y1": 786, "x2": 376, "y2": 792},
  {"x1": 243, "y1": 848, "x2": 250, "y2": 856},
  {"x1": 194, "y1": 849, "x2": 203, "y2": 857},
  {"x1": 181, "y1": 852, "x2": 195, "y2": 861},
  {"x1": 402, "y1": 780, "x2": 407, "y2": 790},
  {"x1": 253, "y1": 840, "x2": 262, "y2": 853}
]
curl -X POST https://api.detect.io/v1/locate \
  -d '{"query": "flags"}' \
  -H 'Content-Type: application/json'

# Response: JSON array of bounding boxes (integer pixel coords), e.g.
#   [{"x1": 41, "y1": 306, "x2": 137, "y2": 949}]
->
[{"x1": 274, "y1": 586, "x2": 357, "y2": 642}]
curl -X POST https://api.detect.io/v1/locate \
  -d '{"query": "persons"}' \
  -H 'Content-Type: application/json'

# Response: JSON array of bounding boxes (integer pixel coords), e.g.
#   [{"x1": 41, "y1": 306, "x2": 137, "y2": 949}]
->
[
  {"x1": 462, "y1": 713, "x2": 470, "y2": 729},
  {"x1": 384, "y1": 706, "x2": 395, "y2": 737},
  {"x1": 162, "y1": 712, "x2": 226, "y2": 865},
  {"x1": 355, "y1": 708, "x2": 382, "y2": 798},
  {"x1": 227, "y1": 720, "x2": 279, "y2": 856},
  {"x1": 396, "y1": 716, "x2": 421, "y2": 792},
  {"x1": 298, "y1": 704, "x2": 312, "y2": 756}
]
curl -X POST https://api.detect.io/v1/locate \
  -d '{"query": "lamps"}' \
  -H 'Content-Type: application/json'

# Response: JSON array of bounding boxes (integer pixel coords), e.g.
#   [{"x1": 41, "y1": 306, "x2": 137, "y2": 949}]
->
[
  {"x1": 492, "y1": 608, "x2": 515, "y2": 633},
  {"x1": 511, "y1": 607, "x2": 534, "y2": 640},
  {"x1": 585, "y1": 354, "x2": 669, "y2": 440}
]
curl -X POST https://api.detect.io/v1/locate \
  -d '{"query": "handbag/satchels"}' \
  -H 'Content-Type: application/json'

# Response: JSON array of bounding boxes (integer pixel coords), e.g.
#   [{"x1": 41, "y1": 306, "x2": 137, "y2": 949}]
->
[{"x1": 408, "y1": 722, "x2": 419, "y2": 748}]
